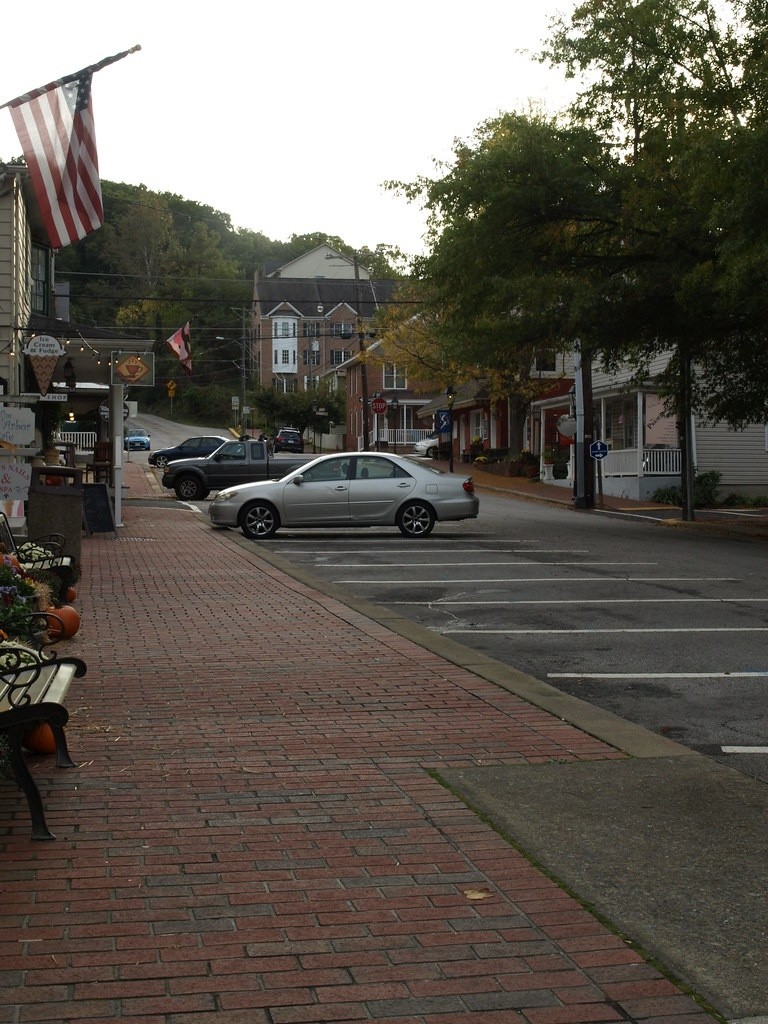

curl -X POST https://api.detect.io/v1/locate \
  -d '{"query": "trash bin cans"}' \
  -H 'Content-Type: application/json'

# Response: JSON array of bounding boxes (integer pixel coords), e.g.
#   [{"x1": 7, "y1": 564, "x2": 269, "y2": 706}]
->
[{"x1": 27, "y1": 465, "x2": 83, "y2": 575}]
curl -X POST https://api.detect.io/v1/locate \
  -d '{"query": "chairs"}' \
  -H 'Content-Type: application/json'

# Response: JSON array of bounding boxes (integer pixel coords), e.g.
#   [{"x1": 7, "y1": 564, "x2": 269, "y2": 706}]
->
[
  {"x1": 342, "y1": 463, "x2": 349, "y2": 474},
  {"x1": 86, "y1": 441, "x2": 113, "y2": 487},
  {"x1": 361, "y1": 468, "x2": 369, "y2": 478}
]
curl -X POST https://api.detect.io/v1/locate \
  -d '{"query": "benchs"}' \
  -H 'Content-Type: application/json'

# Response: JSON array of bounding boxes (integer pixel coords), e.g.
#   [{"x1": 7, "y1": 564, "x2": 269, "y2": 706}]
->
[
  {"x1": 0, "y1": 612, "x2": 88, "y2": 842},
  {"x1": 0, "y1": 512, "x2": 76, "y2": 603}
]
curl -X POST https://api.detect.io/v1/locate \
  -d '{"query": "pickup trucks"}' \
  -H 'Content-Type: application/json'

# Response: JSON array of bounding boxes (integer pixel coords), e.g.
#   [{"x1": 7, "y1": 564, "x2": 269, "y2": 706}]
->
[{"x1": 161, "y1": 435, "x2": 341, "y2": 500}]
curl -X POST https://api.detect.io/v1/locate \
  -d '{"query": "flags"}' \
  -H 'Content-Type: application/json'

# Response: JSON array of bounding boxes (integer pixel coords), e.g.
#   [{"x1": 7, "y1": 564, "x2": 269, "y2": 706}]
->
[
  {"x1": 166, "y1": 323, "x2": 192, "y2": 374},
  {"x1": 9, "y1": 56, "x2": 118, "y2": 250}
]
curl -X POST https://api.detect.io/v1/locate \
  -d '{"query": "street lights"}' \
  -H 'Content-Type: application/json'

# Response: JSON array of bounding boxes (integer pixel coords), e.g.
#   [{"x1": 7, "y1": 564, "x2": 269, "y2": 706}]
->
[
  {"x1": 445, "y1": 384, "x2": 456, "y2": 473},
  {"x1": 391, "y1": 395, "x2": 399, "y2": 454}
]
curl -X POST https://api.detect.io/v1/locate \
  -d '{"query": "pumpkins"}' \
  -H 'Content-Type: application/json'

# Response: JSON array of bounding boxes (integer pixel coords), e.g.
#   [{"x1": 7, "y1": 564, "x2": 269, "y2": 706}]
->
[
  {"x1": 0, "y1": 552, "x2": 18, "y2": 567},
  {"x1": 22, "y1": 722, "x2": 68, "y2": 755},
  {"x1": 65, "y1": 586, "x2": 76, "y2": 601},
  {"x1": 45, "y1": 601, "x2": 80, "y2": 639}
]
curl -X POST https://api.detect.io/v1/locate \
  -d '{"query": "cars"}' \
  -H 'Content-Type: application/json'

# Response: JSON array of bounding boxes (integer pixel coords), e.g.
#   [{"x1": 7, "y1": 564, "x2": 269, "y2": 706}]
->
[
  {"x1": 208, "y1": 452, "x2": 479, "y2": 538},
  {"x1": 413, "y1": 430, "x2": 440, "y2": 457},
  {"x1": 124, "y1": 428, "x2": 151, "y2": 450},
  {"x1": 149, "y1": 435, "x2": 246, "y2": 468}
]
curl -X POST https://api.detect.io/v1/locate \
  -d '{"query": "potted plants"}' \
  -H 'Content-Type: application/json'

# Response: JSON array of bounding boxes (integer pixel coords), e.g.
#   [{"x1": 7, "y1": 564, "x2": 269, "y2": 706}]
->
[
  {"x1": 542, "y1": 449, "x2": 557, "y2": 464},
  {"x1": 526, "y1": 457, "x2": 537, "y2": 478}
]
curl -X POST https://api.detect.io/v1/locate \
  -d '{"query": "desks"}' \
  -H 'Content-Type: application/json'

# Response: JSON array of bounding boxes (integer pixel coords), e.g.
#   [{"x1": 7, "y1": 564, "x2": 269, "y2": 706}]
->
[
  {"x1": 55, "y1": 443, "x2": 76, "y2": 467},
  {"x1": 32, "y1": 466, "x2": 84, "y2": 488}
]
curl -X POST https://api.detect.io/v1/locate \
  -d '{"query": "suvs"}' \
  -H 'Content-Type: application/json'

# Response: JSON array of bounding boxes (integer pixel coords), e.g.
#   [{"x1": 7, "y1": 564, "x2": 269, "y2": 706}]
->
[{"x1": 259, "y1": 426, "x2": 305, "y2": 455}]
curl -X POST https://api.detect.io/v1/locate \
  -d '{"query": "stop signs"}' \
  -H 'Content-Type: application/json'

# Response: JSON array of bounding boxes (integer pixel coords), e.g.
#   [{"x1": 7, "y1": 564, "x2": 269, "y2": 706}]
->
[{"x1": 372, "y1": 398, "x2": 388, "y2": 414}]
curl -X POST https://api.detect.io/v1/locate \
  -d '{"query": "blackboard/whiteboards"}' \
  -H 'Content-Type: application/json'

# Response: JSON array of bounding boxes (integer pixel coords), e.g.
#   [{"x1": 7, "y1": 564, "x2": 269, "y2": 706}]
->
[{"x1": 82, "y1": 482, "x2": 116, "y2": 533}]
